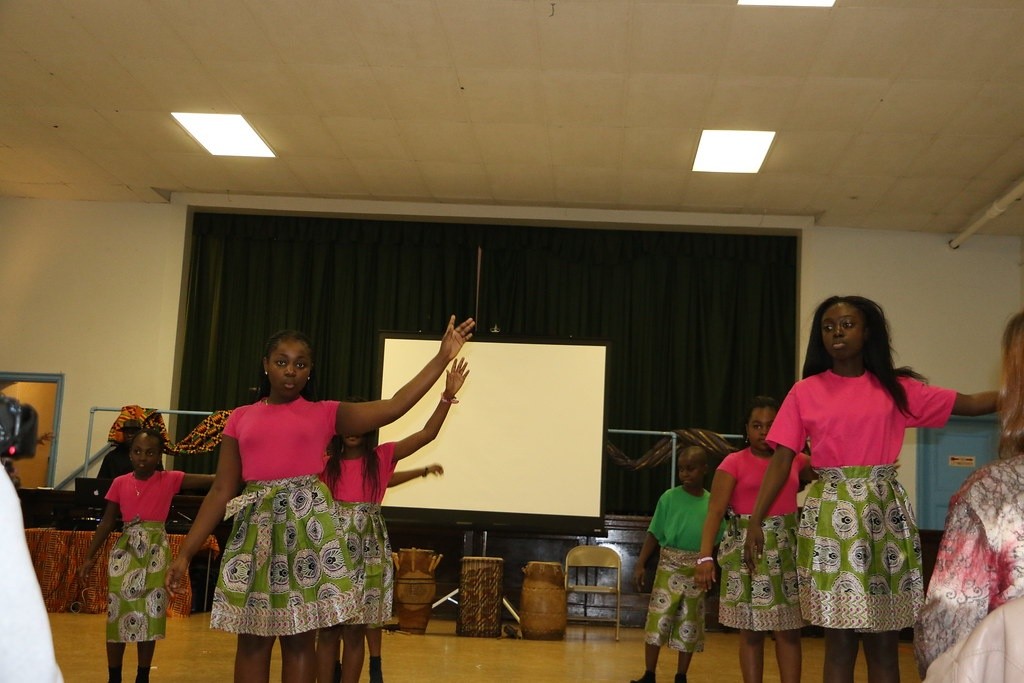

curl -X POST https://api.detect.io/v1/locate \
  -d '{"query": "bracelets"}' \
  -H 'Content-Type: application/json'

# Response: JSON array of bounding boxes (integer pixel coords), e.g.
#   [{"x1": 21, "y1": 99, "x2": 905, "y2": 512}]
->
[
  {"x1": 441, "y1": 391, "x2": 459, "y2": 404},
  {"x1": 422, "y1": 467, "x2": 429, "y2": 477}
]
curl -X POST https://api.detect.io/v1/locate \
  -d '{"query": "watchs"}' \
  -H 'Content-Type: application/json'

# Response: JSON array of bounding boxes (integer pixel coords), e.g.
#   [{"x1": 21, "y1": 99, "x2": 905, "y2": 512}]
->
[{"x1": 697, "y1": 557, "x2": 713, "y2": 564}]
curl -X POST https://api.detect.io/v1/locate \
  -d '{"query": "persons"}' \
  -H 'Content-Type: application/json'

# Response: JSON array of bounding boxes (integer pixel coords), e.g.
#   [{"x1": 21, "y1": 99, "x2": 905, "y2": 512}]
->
[
  {"x1": 163, "y1": 314, "x2": 475, "y2": 683},
  {"x1": 80, "y1": 430, "x2": 217, "y2": 682},
  {"x1": 745, "y1": 295, "x2": 1003, "y2": 683},
  {"x1": 630, "y1": 445, "x2": 726, "y2": 683},
  {"x1": 97, "y1": 418, "x2": 164, "y2": 496},
  {"x1": 694, "y1": 396, "x2": 813, "y2": 683},
  {"x1": 913, "y1": 311, "x2": 1024, "y2": 683},
  {"x1": 315, "y1": 357, "x2": 470, "y2": 683},
  {"x1": 334, "y1": 464, "x2": 444, "y2": 683}
]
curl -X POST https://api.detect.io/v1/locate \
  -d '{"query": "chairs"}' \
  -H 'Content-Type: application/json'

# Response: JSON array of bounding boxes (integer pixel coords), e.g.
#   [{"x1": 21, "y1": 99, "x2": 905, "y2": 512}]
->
[{"x1": 563, "y1": 542, "x2": 623, "y2": 645}]
